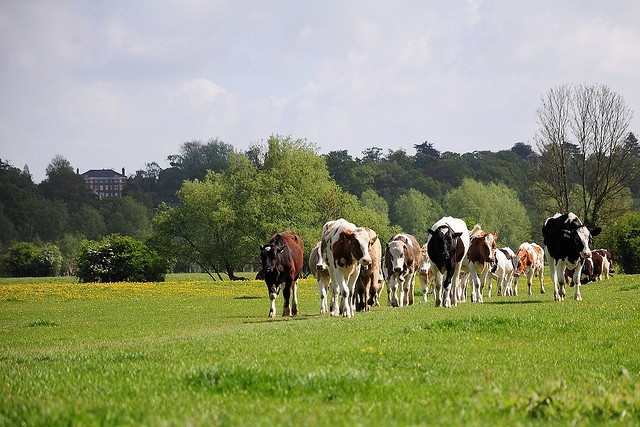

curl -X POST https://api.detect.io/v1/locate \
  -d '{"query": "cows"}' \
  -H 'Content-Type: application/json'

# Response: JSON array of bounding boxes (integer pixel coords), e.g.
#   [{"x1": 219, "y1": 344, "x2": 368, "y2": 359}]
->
[
  {"x1": 383, "y1": 229, "x2": 423, "y2": 309},
  {"x1": 317, "y1": 217, "x2": 374, "y2": 320},
  {"x1": 510, "y1": 241, "x2": 547, "y2": 298},
  {"x1": 462, "y1": 224, "x2": 498, "y2": 303},
  {"x1": 539, "y1": 210, "x2": 602, "y2": 302},
  {"x1": 426, "y1": 214, "x2": 473, "y2": 309},
  {"x1": 487, "y1": 246, "x2": 515, "y2": 297},
  {"x1": 419, "y1": 242, "x2": 436, "y2": 301},
  {"x1": 563, "y1": 247, "x2": 615, "y2": 289},
  {"x1": 309, "y1": 240, "x2": 331, "y2": 314},
  {"x1": 259, "y1": 230, "x2": 306, "y2": 319}
]
